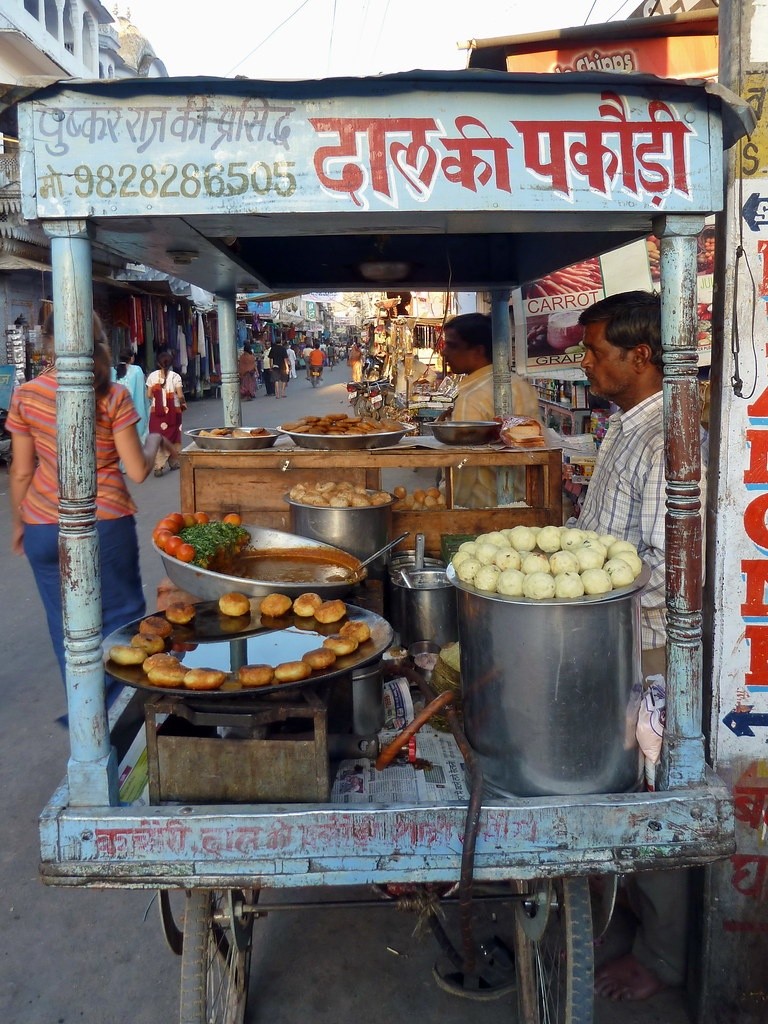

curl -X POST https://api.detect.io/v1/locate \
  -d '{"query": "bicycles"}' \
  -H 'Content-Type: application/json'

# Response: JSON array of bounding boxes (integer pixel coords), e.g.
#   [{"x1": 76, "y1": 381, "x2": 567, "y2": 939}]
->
[{"x1": 329, "y1": 356, "x2": 333, "y2": 370}]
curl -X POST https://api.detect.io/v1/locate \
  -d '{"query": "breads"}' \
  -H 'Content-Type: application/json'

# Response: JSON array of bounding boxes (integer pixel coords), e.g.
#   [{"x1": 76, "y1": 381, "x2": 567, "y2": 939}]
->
[
  {"x1": 108, "y1": 593, "x2": 370, "y2": 689},
  {"x1": 389, "y1": 485, "x2": 448, "y2": 510},
  {"x1": 282, "y1": 413, "x2": 404, "y2": 435},
  {"x1": 502, "y1": 419, "x2": 545, "y2": 448},
  {"x1": 199, "y1": 427, "x2": 271, "y2": 439}
]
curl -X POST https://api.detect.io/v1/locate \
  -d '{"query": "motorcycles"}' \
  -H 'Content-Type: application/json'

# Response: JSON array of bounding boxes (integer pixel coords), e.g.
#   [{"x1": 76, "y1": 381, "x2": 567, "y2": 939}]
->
[{"x1": 347, "y1": 377, "x2": 390, "y2": 417}]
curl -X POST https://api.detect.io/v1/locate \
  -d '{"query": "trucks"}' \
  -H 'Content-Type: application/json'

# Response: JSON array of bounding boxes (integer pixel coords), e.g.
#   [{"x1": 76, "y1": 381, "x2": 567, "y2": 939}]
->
[{"x1": 1, "y1": 69, "x2": 758, "y2": 1024}]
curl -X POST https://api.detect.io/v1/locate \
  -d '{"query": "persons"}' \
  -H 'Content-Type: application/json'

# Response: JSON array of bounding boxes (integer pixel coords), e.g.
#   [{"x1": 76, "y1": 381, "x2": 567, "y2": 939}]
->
[
  {"x1": 237, "y1": 340, "x2": 259, "y2": 401},
  {"x1": 261, "y1": 337, "x2": 351, "y2": 399},
  {"x1": 566, "y1": 291, "x2": 692, "y2": 1005},
  {"x1": 434, "y1": 313, "x2": 541, "y2": 509},
  {"x1": 146, "y1": 352, "x2": 188, "y2": 477},
  {"x1": 6, "y1": 306, "x2": 178, "y2": 727},
  {"x1": 109, "y1": 349, "x2": 150, "y2": 473}
]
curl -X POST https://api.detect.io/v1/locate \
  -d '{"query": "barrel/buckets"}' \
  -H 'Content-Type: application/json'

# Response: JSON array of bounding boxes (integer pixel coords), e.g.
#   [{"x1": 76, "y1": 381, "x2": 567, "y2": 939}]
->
[
  {"x1": 446, "y1": 558, "x2": 652, "y2": 797},
  {"x1": 282, "y1": 490, "x2": 399, "y2": 578},
  {"x1": 388, "y1": 549, "x2": 458, "y2": 647},
  {"x1": 352, "y1": 660, "x2": 385, "y2": 735}
]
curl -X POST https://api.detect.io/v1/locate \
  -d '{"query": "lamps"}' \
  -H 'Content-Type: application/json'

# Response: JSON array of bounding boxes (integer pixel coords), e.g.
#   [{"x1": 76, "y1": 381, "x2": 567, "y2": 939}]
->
[{"x1": 358, "y1": 236, "x2": 410, "y2": 280}]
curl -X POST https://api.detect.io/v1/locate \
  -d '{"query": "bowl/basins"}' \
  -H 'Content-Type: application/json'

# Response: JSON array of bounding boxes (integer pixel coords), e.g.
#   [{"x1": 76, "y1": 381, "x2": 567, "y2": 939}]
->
[
  {"x1": 276, "y1": 422, "x2": 416, "y2": 449},
  {"x1": 152, "y1": 519, "x2": 367, "y2": 601},
  {"x1": 186, "y1": 427, "x2": 284, "y2": 449},
  {"x1": 409, "y1": 641, "x2": 439, "y2": 682},
  {"x1": 423, "y1": 421, "x2": 502, "y2": 445}
]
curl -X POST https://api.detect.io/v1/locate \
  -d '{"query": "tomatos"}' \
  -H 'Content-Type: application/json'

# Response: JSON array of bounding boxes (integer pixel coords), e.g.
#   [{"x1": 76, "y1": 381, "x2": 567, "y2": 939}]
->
[
  {"x1": 153, "y1": 511, "x2": 209, "y2": 563},
  {"x1": 223, "y1": 513, "x2": 242, "y2": 527}
]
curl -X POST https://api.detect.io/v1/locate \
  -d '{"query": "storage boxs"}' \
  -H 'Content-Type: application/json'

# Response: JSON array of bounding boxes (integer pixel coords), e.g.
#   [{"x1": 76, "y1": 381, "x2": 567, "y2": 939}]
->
[
  {"x1": 571, "y1": 381, "x2": 590, "y2": 408},
  {"x1": 564, "y1": 432, "x2": 599, "y2": 477}
]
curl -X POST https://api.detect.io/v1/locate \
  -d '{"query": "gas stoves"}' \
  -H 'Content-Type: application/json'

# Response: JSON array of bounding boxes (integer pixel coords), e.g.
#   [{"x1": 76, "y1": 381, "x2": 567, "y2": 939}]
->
[{"x1": 143, "y1": 696, "x2": 417, "y2": 806}]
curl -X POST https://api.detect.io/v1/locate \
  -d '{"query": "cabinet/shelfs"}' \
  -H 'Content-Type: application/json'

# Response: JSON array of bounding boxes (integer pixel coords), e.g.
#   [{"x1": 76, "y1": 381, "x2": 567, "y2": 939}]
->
[{"x1": 532, "y1": 380, "x2": 592, "y2": 436}]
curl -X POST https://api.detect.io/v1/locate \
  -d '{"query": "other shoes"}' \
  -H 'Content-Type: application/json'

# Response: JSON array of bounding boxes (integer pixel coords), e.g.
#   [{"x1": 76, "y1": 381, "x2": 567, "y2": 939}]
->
[
  {"x1": 154, "y1": 468, "x2": 163, "y2": 477},
  {"x1": 276, "y1": 396, "x2": 279, "y2": 399},
  {"x1": 264, "y1": 394, "x2": 275, "y2": 396},
  {"x1": 169, "y1": 462, "x2": 181, "y2": 471},
  {"x1": 281, "y1": 395, "x2": 287, "y2": 398},
  {"x1": 432, "y1": 935, "x2": 558, "y2": 1003},
  {"x1": 318, "y1": 375, "x2": 323, "y2": 381}
]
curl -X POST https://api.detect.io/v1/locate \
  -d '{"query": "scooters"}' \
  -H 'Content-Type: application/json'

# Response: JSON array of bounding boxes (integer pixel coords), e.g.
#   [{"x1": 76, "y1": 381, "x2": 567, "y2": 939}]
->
[{"x1": 310, "y1": 366, "x2": 321, "y2": 387}]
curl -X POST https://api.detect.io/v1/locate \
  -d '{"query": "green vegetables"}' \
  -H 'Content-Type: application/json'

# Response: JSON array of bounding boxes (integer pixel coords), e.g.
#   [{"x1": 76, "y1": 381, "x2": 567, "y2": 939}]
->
[{"x1": 176, "y1": 521, "x2": 250, "y2": 568}]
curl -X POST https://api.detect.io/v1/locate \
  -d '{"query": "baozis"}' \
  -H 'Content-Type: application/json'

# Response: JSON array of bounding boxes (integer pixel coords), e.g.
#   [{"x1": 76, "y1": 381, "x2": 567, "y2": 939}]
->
[
  {"x1": 289, "y1": 481, "x2": 392, "y2": 508},
  {"x1": 451, "y1": 524, "x2": 643, "y2": 600}
]
